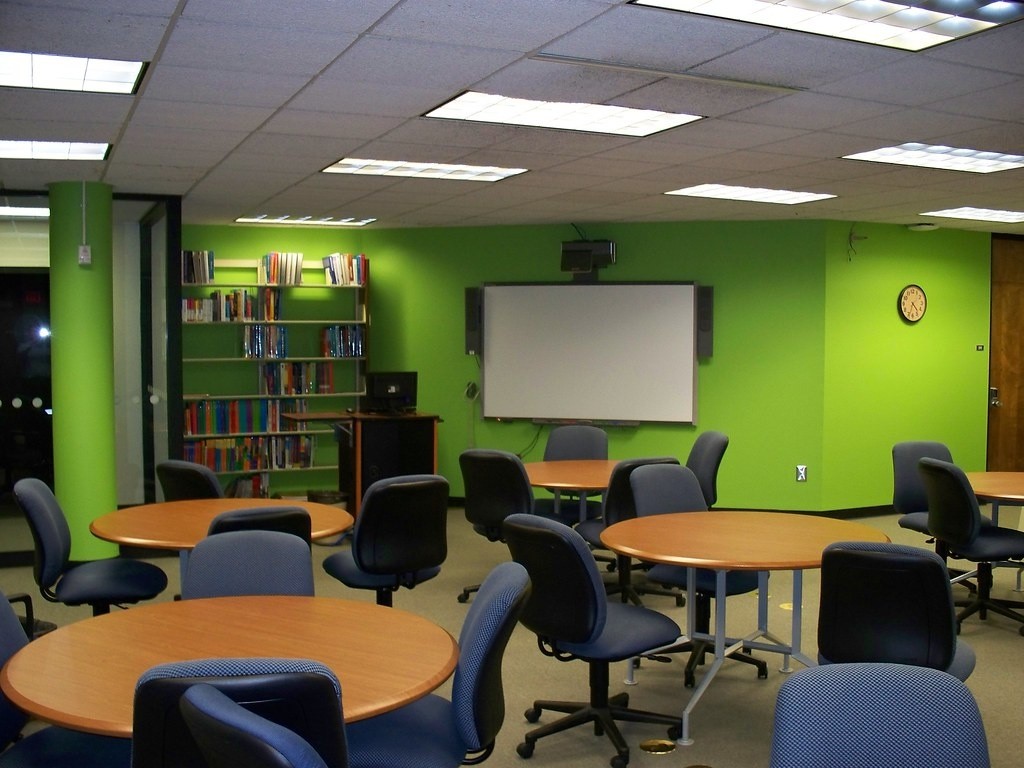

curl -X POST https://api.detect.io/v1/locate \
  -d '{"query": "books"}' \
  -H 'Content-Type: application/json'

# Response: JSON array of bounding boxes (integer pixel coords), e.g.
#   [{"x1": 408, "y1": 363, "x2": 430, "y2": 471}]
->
[{"x1": 180, "y1": 250, "x2": 364, "y2": 504}]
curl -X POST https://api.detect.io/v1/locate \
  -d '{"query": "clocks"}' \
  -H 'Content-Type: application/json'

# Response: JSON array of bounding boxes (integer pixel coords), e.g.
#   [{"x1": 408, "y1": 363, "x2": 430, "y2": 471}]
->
[{"x1": 898, "y1": 285, "x2": 927, "y2": 323}]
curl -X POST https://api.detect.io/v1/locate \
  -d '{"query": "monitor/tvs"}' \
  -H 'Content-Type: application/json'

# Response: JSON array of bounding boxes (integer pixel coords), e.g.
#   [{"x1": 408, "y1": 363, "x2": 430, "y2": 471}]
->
[{"x1": 360, "y1": 371, "x2": 418, "y2": 416}]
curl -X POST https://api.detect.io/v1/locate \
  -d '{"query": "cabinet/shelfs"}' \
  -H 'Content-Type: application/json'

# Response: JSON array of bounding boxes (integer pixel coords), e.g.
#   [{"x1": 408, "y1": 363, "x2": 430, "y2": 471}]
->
[{"x1": 183, "y1": 250, "x2": 370, "y2": 502}]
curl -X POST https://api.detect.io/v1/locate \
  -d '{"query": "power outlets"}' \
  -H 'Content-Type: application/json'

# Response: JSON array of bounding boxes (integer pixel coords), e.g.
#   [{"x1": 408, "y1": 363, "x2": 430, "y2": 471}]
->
[{"x1": 796, "y1": 466, "x2": 806, "y2": 481}]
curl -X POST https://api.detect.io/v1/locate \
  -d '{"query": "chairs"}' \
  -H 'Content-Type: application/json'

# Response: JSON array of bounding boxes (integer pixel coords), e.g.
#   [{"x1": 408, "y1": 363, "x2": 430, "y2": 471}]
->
[{"x1": 0, "y1": 424, "x2": 1024, "y2": 768}]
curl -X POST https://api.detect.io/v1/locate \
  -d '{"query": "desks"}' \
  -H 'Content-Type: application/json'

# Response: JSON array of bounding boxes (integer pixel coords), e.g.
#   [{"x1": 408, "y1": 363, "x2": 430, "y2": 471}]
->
[
  {"x1": 964, "y1": 472, "x2": 1024, "y2": 593},
  {"x1": 90, "y1": 497, "x2": 355, "y2": 549},
  {"x1": 523, "y1": 460, "x2": 621, "y2": 521},
  {"x1": 282, "y1": 412, "x2": 441, "y2": 530},
  {"x1": 600, "y1": 511, "x2": 893, "y2": 750},
  {"x1": 0, "y1": 593, "x2": 459, "y2": 738}
]
